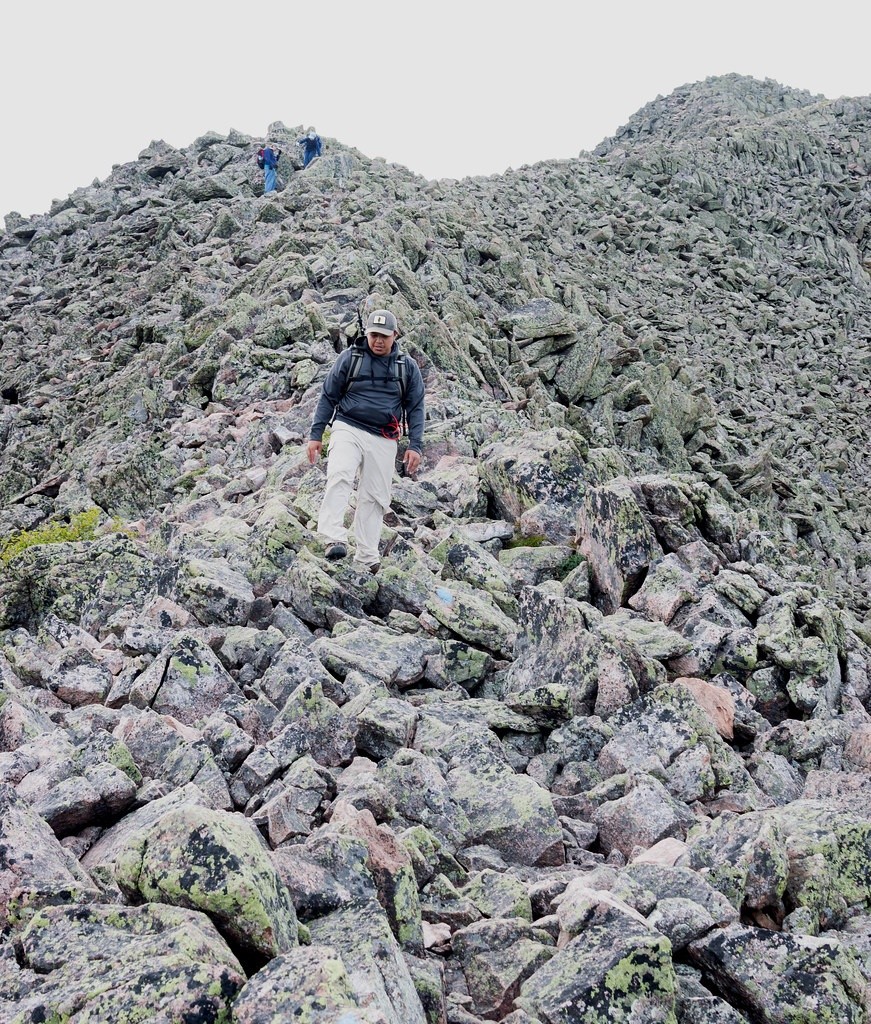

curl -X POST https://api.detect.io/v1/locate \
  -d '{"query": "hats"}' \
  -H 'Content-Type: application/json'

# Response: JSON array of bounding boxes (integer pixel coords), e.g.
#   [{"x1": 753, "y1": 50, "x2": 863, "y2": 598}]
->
[
  {"x1": 272, "y1": 143, "x2": 281, "y2": 151},
  {"x1": 309, "y1": 132, "x2": 316, "y2": 139},
  {"x1": 364, "y1": 309, "x2": 398, "y2": 336}
]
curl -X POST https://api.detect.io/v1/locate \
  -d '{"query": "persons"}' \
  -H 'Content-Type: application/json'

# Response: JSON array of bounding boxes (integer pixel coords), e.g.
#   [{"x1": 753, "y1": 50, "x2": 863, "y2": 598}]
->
[
  {"x1": 263, "y1": 143, "x2": 280, "y2": 193},
  {"x1": 300, "y1": 132, "x2": 322, "y2": 169},
  {"x1": 306, "y1": 310, "x2": 424, "y2": 572}
]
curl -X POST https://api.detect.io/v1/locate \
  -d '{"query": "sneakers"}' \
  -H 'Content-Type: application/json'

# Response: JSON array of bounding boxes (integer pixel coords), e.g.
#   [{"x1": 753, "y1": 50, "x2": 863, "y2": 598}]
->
[
  {"x1": 324, "y1": 543, "x2": 346, "y2": 559},
  {"x1": 369, "y1": 562, "x2": 381, "y2": 573}
]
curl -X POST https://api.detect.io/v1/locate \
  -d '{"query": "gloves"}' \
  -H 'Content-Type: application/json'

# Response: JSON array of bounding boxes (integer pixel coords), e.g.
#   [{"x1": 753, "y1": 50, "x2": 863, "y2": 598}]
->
[
  {"x1": 273, "y1": 164, "x2": 278, "y2": 169},
  {"x1": 279, "y1": 150, "x2": 282, "y2": 155}
]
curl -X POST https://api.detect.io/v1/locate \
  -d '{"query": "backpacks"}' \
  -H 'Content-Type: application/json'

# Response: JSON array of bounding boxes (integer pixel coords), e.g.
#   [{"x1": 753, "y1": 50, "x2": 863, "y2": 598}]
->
[{"x1": 257, "y1": 148, "x2": 273, "y2": 169}]
